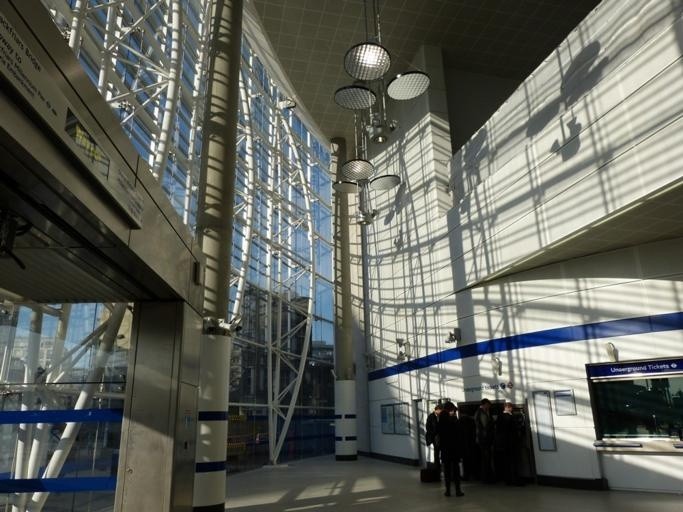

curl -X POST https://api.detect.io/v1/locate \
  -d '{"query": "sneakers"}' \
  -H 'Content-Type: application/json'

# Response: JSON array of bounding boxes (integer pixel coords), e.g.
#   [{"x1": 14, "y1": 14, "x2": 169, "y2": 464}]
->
[
  {"x1": 444, "y1": 491, "x2": 451, "y2": 498},
  {"x1": 456, "y1": 492, "x2": 464, "y2": 497}
]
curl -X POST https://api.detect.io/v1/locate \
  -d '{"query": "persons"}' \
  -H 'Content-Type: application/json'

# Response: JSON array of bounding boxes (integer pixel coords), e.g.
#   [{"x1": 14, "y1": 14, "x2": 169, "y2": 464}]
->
[{"x1": 425, "y1": 399, "x2": 525, "y2": 497}]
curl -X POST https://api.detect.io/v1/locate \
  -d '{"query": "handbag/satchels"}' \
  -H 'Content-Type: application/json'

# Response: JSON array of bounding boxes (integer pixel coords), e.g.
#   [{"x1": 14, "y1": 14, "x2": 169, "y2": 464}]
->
[{"x1": 419, "y1": 462, "x2": 442, "y2": 483}]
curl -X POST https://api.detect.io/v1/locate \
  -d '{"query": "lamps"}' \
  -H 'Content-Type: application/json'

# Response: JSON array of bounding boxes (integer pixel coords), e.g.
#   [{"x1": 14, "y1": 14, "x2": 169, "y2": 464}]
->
[{"x1": 329, "y1": 1, "x2": 431, "y2": 195}]
